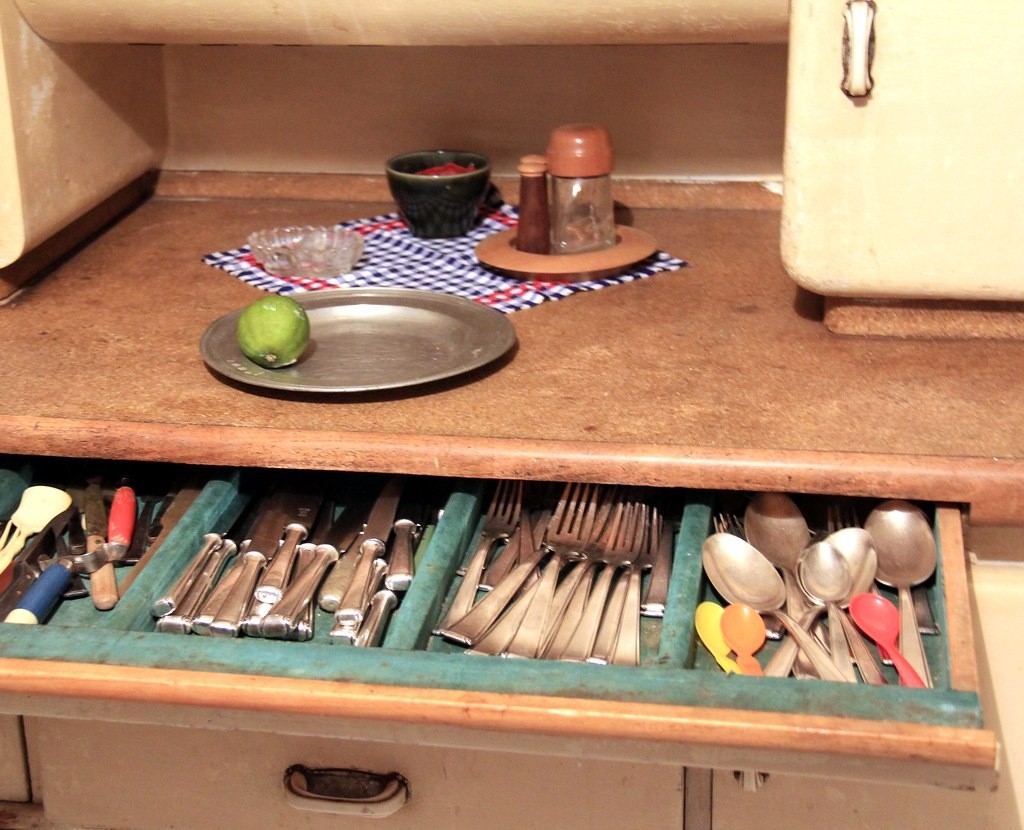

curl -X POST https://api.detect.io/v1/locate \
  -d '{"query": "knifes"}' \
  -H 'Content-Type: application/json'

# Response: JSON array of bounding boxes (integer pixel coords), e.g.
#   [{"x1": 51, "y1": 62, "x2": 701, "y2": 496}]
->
[
  {"x1": 83, "y1": 484, "x2": 118, "y2": 609},
  {"x1": 150, "y1": 479, "x2": 426, "y2": 648}
]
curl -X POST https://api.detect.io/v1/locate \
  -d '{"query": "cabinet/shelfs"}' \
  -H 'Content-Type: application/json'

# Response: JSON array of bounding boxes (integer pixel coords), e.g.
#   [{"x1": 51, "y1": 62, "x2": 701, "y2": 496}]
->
[{"x1": 0, "y1": 0, "x2": 1024, "y2": 830}]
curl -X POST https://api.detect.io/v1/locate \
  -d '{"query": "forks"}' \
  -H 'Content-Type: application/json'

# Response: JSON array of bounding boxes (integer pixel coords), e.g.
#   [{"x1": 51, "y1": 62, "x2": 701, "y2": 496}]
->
[
  {"x1": 711, "y1": 511, "x2": 747, "y2": 542},
  {"x1": 826, "y1": 505, "x2": 895, "y2": 665},
  {"x1": 429, "y1": 480, "x2": 673, "y2": 668}
]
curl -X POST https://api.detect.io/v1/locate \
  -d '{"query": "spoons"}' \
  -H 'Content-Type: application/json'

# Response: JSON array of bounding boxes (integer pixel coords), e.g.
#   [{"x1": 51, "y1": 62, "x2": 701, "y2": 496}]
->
[{"x1": 694, "y1": 491, "x2": 939, "y2": 690}]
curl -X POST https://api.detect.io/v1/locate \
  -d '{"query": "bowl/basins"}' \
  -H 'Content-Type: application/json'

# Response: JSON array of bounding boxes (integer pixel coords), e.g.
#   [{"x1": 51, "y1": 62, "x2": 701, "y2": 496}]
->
[{"x1": 246, "y1": 227, "x2": 361, "y2": 277}]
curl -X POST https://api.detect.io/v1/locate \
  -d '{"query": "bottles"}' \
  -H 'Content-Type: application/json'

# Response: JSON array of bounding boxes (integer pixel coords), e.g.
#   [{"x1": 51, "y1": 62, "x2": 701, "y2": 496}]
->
[
  {"x1": 550, "y1": 122, "x2": 612, "y2": 252},
  {"x1": 516, "y1": 154, "x2": 550, "y2": 251}
]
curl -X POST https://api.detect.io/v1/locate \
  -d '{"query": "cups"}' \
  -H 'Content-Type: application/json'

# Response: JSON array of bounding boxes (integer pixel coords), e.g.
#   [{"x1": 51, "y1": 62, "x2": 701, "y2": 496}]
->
[{"x1": 386, "y1": 150, "x2": 485, "y2": 236}]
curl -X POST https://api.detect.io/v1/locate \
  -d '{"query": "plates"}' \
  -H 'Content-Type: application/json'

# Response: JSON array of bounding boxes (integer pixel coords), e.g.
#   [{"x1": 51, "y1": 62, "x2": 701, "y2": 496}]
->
[
  {"x1": 474, "y1": 221, "x2": 655, "y2": 282},
  {"x1": 197, "y1": 287, "x2": 516, "y2": 392}
]
